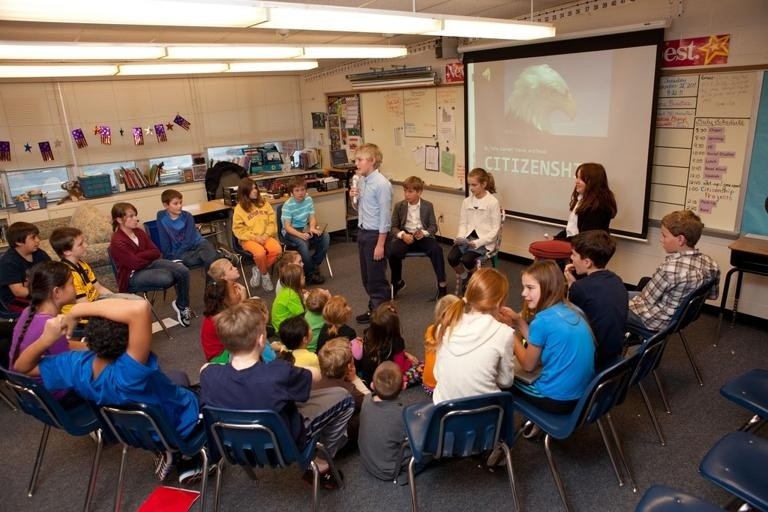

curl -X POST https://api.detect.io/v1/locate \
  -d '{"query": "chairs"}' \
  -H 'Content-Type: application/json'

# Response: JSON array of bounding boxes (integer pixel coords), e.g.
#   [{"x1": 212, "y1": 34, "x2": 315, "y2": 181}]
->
[
  {"x1": 699, "y1": 431, "x2": 768, "y2": 512},
  {"x1": 402, "y1": 391, "x2": 523, "y2": 512},
  {"x1": 0, "y1": 367, "x2": 102, "y2": 512},
  {"x1": 208, "y1": 170, "x2": 241, "y2": 199},
  {"x1": 636, "y1": 485, "x2": 729, "y2": 512},
  {"x1": 390, "y1": 250, "x2": 444, "y2": 300},
  {"x1": 202, "y1": 405, "x2": 343, "y2": 511},
  {"x1": 720, "y1": 368, "x2": 768, "y2": 432},
  {"x1": 97, "y1": 403, "x2": 208, "y2": 512},
  {"x1": 630, "y1": 278, "x2": 715, "y2": 386},
  {"x1": 512, "y1": 352, "x2": 641, "y2": 512},
  {"x1": 626, "y1": 317, "x2": 678, "y2": 446}
]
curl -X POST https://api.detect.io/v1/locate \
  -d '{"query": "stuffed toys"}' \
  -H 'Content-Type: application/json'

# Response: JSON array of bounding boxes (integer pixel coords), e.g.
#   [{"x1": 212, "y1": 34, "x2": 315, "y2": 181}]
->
[{"x1": 57, "y1": 180, "x2": 86, "y2": 205}]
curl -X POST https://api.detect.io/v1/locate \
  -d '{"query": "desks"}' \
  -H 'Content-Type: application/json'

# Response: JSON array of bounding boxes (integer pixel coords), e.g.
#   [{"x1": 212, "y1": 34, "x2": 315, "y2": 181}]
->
[
  {"x1": 713, "y1": 234, "x2": 768, "y2": 347},
  {"x1": 182, "y1": 187, "x2": 349, "y2": 253}
]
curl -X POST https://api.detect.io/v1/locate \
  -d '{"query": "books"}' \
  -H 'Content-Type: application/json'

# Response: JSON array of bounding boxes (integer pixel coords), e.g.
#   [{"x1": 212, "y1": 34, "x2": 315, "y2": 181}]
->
[
  {"x1": 113, "y1": 163, "x2": 158, "y2": 191},
  {"x1": 134, "y1": 481, "x2": 201, "y2": 511}
]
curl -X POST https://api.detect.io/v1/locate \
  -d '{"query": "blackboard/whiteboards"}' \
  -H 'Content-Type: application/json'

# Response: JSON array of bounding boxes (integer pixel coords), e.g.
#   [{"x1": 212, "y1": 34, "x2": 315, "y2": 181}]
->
[{"x1": 359, "y1": 64, "x2": 765, "y2": 241}]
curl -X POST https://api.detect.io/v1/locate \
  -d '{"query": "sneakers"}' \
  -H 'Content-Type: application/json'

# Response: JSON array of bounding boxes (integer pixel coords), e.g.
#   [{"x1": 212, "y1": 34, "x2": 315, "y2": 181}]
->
[
  {"x1": 175, "y1": 458, "x2": 217, "y2": 487},
  {"x1": 436, "y1": 280, "x2": 447, "y2": 301},
  {"x1": 356, "y1": 311, "x2": 372, "y2": 324},
  {"x1": 219, "y1": 246, "x2": 239, "y2": 265},
  {"x1": 261, "y1": 271, "x2": 274, "y2": 293},
  {"x1": 460, "y1": 272, "x2": 473, "y2": 295},
  {"x1": 305, "y1": 273, "x2": 326, "y2": 284},
  {"x1": 172, "y1": 300, "x2": 191, "y2": 327},
  {"x1": 250, "y1": 266, "x2": 261, "y2": 288},
  {"x1": 394, "y1": 280, "x2": 407, "y2": 297},
  {"x1": 302, "y1": 468, "x2": 344, "y2": 490}
]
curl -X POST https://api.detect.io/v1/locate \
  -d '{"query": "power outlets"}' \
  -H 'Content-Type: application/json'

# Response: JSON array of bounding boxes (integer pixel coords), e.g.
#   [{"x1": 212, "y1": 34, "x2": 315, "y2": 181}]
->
[{"x1": 439, "y1": 214, "x2": 443, "y2": 223}]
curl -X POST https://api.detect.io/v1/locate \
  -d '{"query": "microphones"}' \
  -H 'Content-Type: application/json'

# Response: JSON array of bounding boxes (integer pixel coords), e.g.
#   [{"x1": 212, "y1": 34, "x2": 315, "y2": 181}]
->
[{"x1": 352, "y1": 174, "x2": 360, "y2": 205}]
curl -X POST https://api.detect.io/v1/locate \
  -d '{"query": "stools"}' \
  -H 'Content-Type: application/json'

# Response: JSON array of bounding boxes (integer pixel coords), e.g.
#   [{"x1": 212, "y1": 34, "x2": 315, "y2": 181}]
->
[{"x1": 522, "y1": 240, "x2": 571, "y2": 319}]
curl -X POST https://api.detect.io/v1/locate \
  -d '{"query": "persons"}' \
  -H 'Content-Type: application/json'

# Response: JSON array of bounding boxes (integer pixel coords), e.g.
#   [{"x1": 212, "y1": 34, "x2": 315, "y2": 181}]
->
[
  {"x1": 628, "y1": 210, "x2": 719, "y2": 333},
  {"x1": 388, "y1": 176, "x2": 447, "y2": 301},
  {"x1": 157, "y1": 189, "x2": 239, "y2": 288},
  {"x1": 49, "y1": 227, "x2": 114, "y2": 325},
  {"x1": 0, "y1": 222, "x2": 52, "y2": 319},
  {"x1": 110, "y1": 202, "x2": 192, "y2": 328},
  {"x1": 200, "y1": 251, "x2": 422, "y2": 493},
  {"x1": 13, "y1": 293, "x2": 217, "y2": 484},
  {"x1": 432, "y1": 268, "x2": 514, "y2": 468},
  {"x1": 231, "y1": 177, "x2": 282, "y2": 293},
  {"x1": 280, "y1": 175, "x2": 330, "y2": 285},
  {"x1": 422, "y1": 294, "x2": 463, "y2": 398},
  {"x1": 9, "y1": 260, "x2": 86, "y2": 411},
  {"x1": 496, "y1": 259, "x2": 596, "y2": 442},
  {"x1": 553, "y1": 163, "x2": 617, "y2": 273},
  {"x1": 446, "y1": 168, "x2": 501, "y2": 296},
  {"x1": 348, "y1": 143, "x2": 392, "y2": 324},
  {"x1": 564, "y1": 231, "x2": 628, "y2": 373}
]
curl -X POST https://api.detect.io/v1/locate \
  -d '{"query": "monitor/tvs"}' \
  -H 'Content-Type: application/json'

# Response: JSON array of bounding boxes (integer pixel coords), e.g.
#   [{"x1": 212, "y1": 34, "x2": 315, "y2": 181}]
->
[{"x1": 329, "y1": 149, "x2": 349, "y2": 166}]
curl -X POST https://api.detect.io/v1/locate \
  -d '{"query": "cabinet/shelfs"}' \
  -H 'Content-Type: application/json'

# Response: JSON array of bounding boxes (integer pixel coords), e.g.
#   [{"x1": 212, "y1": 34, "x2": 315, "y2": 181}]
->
[{"x1": 327, "y1": 168, "x2": 358, "y2": 222}]
